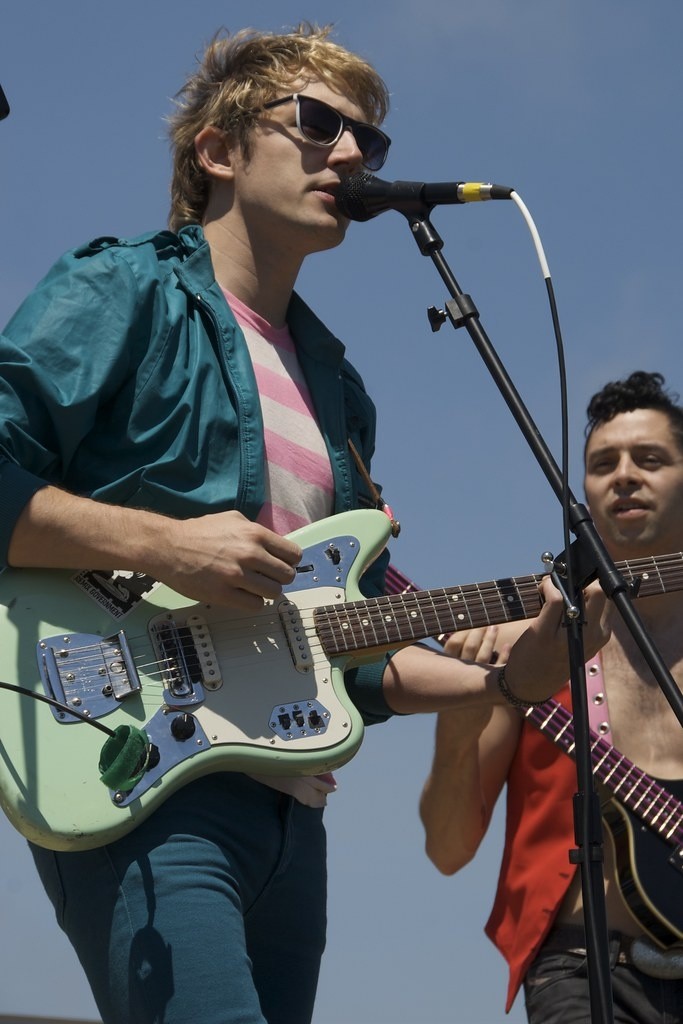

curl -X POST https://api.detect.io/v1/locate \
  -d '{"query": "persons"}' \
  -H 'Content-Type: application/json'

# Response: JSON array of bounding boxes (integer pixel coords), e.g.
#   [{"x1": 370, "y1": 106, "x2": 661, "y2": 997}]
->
[
  {"x1": 419, "y1": 370, "x2": 683, "y2": 1024},
  {"x1": 1, "y1": 20, "x2": 622, "y2": 1023}
]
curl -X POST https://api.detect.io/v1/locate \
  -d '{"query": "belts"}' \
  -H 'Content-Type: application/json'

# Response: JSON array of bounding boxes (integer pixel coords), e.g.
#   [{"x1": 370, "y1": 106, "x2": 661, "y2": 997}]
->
[{"x1": 566, "y1": 930, "x2": 683, "y2": 980}]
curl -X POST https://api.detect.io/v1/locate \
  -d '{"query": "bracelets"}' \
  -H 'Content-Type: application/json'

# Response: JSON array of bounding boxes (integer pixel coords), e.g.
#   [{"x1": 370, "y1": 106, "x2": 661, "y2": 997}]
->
[{"x1": 499, "y1": 667, "x2": 550, "y2": 708}]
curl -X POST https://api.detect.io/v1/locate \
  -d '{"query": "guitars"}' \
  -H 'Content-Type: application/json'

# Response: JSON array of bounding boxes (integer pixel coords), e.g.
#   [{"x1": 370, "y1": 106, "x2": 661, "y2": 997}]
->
[
  {"x1": 381, "y1": 558, "x2": 683, "y2": 951},
  {"x1": 0, "y1": 504, "x2": 683, "y2": 851}
]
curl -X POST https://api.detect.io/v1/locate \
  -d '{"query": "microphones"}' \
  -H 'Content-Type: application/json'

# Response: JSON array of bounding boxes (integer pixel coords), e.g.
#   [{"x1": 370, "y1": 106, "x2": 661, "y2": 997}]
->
[{"x1": 335, "y1": 171, "x2": 516, "y2": 221}]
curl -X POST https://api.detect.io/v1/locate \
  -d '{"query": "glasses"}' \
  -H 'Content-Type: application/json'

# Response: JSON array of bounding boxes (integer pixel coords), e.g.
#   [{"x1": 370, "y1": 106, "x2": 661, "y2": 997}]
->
[{"x1": 228, "y1": 93, "x2": 391, "y2": 171}]
